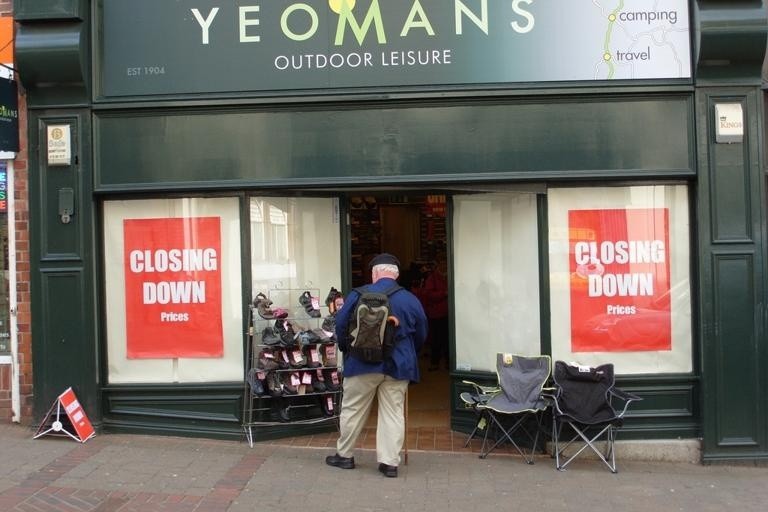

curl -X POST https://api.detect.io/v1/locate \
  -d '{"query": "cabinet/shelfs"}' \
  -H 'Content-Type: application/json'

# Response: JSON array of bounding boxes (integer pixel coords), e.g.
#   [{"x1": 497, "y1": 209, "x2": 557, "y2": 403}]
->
[
  {"x1": 351, "y1": 208, "x2": 383, "y2": 288},
  {"x1": 417, "y1": 206, "x2": 447, "y2": 260},
  {"x1": 239, "y1": 290, "x2": 343, "y2": 449}
]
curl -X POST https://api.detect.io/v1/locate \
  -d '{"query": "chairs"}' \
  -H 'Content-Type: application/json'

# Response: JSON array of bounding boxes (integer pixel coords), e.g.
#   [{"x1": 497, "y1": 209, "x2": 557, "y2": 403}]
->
[
  {"x1": 551, "y1": 360, "x2": 644, "y2": 473},
  {"x1": 472, "y1": 352, "x2": 551, "y2": 465}
]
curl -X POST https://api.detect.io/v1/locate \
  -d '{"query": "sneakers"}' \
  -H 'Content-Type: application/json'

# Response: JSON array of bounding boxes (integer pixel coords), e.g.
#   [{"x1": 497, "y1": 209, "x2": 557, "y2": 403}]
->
[
  {"x1": 378, "y1": 463, "x2": 398, "y2": 477},
  {"x1": 274, "y1": 399, "x2": 291, "y2": 423},
  {"x1": 325, "y1": 453, "x2": 355, "y2": 469},
  {"x1": 325, "y1": 287, "x2": 344, "y2": 317},
  {"x1": 320, "y1": 395, "x2": 333, "y2": 417},
  {"x1": 247, "y1": 319, "x2": 340, "y2": 397}
]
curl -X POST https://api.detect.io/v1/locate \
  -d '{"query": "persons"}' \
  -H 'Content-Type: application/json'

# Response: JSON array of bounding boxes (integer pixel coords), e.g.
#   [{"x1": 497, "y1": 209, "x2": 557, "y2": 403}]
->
[{"x1": 322, "y1": 252, "x2": 430, "y2": 478}]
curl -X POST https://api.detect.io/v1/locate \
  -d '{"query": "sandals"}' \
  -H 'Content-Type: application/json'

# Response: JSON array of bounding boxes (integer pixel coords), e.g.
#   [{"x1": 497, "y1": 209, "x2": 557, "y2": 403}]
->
[
  {"x1": 299, "y1": 291, "x2": 321, "y2": 318},
  {"x1": 253, "y1": 292, "x2": 288, "y2": 319}
]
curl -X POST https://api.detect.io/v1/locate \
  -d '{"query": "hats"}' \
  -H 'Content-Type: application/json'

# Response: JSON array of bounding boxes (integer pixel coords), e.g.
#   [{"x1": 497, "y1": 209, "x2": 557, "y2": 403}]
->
[{"x1": 368, "y1": 253, "x2": 401, "y2": 270}]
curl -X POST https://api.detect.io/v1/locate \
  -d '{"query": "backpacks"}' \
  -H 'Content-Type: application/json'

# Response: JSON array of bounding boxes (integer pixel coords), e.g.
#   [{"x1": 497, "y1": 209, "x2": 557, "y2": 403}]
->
[{"x1": 345, "y1": 284, "x2": 404, "y2": 364}]
